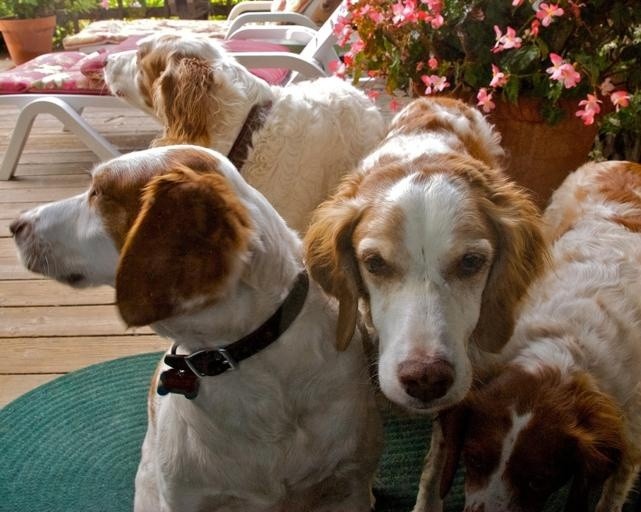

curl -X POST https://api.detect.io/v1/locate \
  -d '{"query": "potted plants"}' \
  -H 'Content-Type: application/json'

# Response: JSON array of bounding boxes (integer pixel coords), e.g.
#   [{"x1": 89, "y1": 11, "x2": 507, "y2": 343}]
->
[{"x1": 0, "y1": 0, "x2": 57, "y2": 66}]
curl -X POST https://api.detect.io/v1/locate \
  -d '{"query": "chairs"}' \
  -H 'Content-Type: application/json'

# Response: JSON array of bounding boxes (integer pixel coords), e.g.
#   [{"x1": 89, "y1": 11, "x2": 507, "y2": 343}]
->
[{"x1": 0, "y1": 0, "x2": 361, "y2": 181}]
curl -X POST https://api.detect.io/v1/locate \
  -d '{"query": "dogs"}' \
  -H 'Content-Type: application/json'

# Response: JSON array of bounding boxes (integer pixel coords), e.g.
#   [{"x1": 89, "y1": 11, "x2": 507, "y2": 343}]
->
[{"x1": 7, "y1": 28, "x2": 641, "y2": 512}]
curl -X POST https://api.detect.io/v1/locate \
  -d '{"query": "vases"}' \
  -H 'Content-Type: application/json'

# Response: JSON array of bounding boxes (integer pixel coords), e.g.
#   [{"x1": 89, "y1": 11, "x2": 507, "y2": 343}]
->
[{"x1": 421, "y1": 93, "x2": 601, "y2": 215}]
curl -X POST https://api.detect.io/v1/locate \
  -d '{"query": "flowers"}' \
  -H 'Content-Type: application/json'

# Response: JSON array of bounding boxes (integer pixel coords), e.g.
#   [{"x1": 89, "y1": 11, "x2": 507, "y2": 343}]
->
[{"x1": 328, "y1": 0, "x2": 641, "y2": 129}]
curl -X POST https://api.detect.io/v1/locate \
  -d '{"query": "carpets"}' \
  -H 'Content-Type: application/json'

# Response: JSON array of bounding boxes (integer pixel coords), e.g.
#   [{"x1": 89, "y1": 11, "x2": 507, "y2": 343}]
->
[{"x1": 1, "y1": 350, "x2": 641, "y2": 512}]
